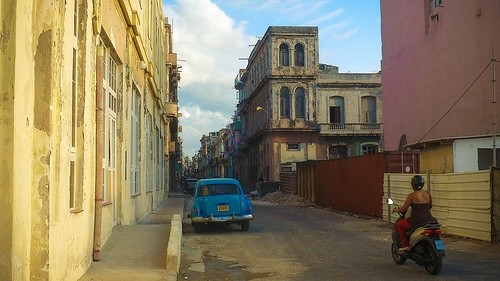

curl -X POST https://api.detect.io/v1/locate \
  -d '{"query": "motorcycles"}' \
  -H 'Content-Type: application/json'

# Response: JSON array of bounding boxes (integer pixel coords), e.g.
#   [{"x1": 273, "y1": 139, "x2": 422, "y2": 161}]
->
[{"x1": 387, "y1": 197, "x2": 447, "y2": 276}]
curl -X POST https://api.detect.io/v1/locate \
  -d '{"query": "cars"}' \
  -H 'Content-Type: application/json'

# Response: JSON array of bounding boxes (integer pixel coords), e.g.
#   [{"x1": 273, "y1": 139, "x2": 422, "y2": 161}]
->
[
  {"x1": 183, "y1": 179, "x2": 198, "y2": 195},
  {"x1": 188, "y1": 177, "x2": 255, "y2": 233}
]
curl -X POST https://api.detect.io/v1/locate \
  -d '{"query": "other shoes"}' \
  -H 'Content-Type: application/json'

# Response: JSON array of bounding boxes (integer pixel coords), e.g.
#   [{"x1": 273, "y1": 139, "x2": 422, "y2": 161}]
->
[{"x1": 397, "y1": 246, "x2": 412, "y2": 251}]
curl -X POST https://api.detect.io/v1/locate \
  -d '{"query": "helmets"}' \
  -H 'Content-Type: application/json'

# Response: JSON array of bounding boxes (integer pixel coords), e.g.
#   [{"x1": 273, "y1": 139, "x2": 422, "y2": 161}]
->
[{"x1": 411, "y1": 174, "x2": 425, "y2": 190}]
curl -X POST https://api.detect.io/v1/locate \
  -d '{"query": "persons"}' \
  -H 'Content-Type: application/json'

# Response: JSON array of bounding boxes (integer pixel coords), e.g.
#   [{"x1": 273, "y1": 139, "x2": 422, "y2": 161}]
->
[{"x1": 393, "y1": 175, "x2": 436, "y2": 250}]
